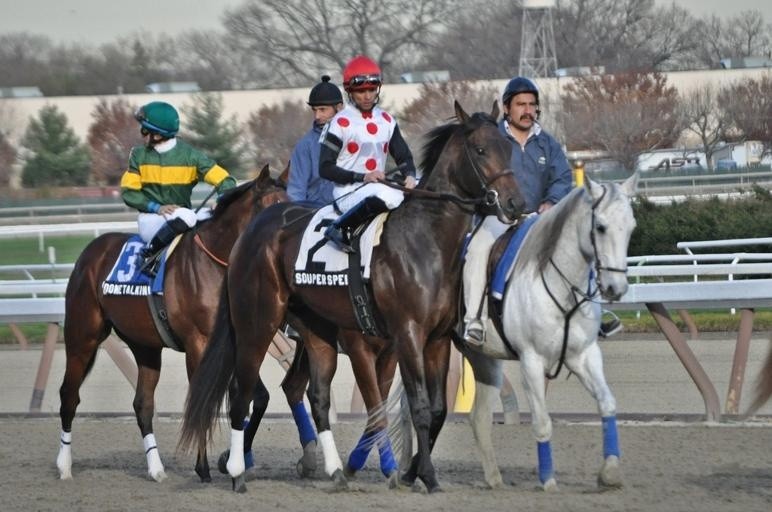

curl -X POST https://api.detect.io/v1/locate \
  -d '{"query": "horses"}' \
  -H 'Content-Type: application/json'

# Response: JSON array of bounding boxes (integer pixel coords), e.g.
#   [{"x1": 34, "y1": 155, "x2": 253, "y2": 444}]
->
[
  {"x1": 358, "y1": 172, "x2": 641, "y2": 494},
  {"x1": 56, "y1": 161, "x2": 290, "y2": 483},
  {"x1": 176, "y1": 100, "x2": 527, "y2": 492}
]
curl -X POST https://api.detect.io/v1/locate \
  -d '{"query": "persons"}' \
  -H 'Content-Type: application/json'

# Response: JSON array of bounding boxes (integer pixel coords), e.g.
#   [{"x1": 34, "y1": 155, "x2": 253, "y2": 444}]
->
[
  {"x1": 286, "y1": 76, "x2": 345, "y2": 205},
  {"x1": 118, "y1": 102, "x2": 237, "y2": 278},
  {"x1": 461, "y1": 78, "x2": 620, "y2": 347},
  {"x1": 316, "y1": 56, "x2": 417, "y2": 254}
]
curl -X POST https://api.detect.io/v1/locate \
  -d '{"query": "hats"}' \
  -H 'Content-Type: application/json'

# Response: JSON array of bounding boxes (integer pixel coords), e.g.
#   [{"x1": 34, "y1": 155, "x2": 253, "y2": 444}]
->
[
  {"x1": 136, "y1": 102, "x2": 180, "y2": 137},
  {"x1": 502, "y1": 77, "x2": 540, "y2": 103},
  {"x1": 343, "y1": 55, "x2": 382, "y2": 91},
  {"x1": 306, "y1": 76, "x2": 343, "y2": 105}
]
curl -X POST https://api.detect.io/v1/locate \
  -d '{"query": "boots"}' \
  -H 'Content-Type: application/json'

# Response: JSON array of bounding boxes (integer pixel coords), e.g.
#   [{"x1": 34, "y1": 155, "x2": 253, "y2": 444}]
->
[
  {"x1": 140, "y1": 222, "x2": 175, "y2": 256},
  {"x1": 324, "y1": 195, "x2": 389, "y2": 255}
]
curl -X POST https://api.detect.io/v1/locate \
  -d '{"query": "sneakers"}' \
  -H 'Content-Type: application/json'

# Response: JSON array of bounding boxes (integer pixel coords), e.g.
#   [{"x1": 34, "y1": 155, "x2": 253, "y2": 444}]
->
[{"x1": 287, "y1": 325, "x2": 302, "y2": 341}]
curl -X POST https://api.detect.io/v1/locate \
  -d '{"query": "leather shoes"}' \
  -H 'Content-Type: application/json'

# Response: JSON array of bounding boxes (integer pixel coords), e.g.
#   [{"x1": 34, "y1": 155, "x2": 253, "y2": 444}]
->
[
  {"x1": 467, "y1": 329, "x2": 483, "y2": 341},
  {"x1": 598, "y1": 320, "x2": 620, "y2": 338}
]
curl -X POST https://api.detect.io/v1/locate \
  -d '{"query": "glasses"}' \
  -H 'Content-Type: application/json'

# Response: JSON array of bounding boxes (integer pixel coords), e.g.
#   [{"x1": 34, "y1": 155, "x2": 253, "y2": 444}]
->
[
  {"x1": 134, "y1": 105, "x2": 145, "y2": 122},
  {"x1": 349, "y1": 75, "x2": 382, "y2": 86}
]
